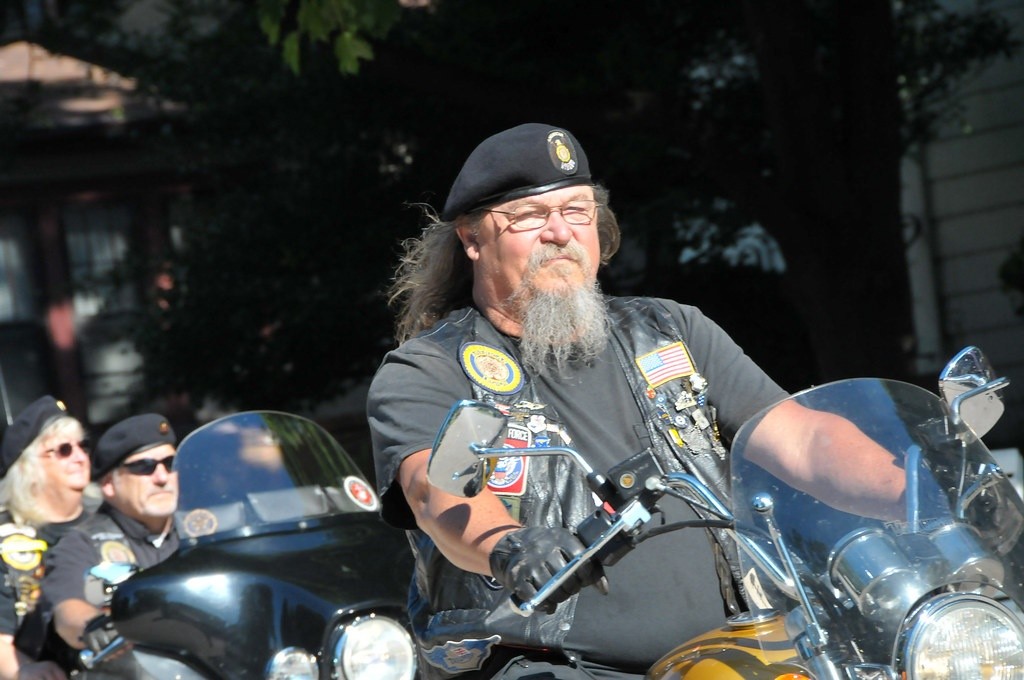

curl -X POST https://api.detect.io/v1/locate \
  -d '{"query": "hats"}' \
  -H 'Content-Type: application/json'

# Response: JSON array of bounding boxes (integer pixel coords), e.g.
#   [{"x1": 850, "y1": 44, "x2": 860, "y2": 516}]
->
[
  {"x1": 0, "y1": 395, "x2": 69, "y2": 478},
  {"x1": 90, "y1": 413, "x2": 177, "y2": 480},
  {"x1": 444, "y1": 124, "x2": 592, "y2": 217}
]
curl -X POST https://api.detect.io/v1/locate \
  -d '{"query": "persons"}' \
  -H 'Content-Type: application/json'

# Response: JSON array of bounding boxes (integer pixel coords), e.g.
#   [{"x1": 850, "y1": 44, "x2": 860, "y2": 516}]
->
[
  {"x1": 364, "y1": 123, "x2": 1024, "y2": 680},
  {"x1": 0, "y1": 395, "x2": 186, "y2": 680}
]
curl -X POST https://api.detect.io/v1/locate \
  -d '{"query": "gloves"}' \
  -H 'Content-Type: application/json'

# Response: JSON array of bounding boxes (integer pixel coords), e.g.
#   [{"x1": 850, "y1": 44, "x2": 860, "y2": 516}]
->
[
  {"x1": 489, "y1": 524, "x2": 608, "y2": 615},
  {"x1": 914, "y1": 462, "x2": 1023, "y2": 554}
]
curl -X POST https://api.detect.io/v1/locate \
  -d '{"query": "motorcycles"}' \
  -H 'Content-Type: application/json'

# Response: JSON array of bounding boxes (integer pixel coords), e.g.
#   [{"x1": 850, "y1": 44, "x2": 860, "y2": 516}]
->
[{"x1": 57, "y1": 346, "x2": 1024, "y2": 680}]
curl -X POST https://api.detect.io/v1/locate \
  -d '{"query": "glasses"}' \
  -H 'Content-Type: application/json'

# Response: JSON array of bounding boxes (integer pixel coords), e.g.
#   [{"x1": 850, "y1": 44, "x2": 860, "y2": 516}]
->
[
  {"x1": 41, "y1": 438, "x2": 91, "y2": 459},
  {"x1": 121, "y1": 454, "x2": 176, "y2": 476},
  {"x1": 480, "y1": 200, "x2": 602, "y2": 230}
]
